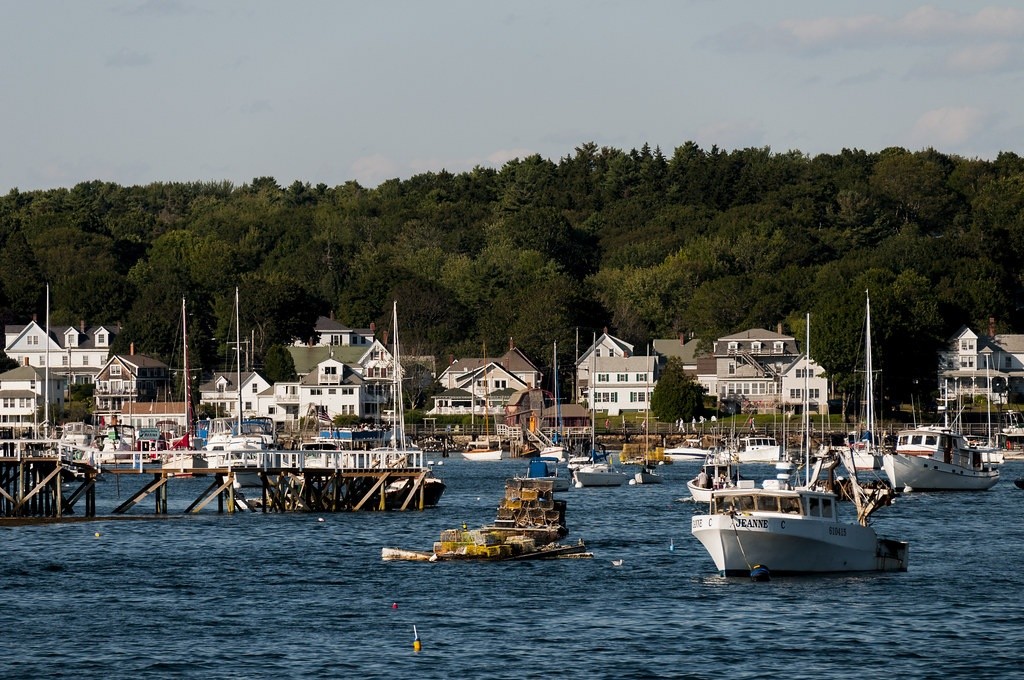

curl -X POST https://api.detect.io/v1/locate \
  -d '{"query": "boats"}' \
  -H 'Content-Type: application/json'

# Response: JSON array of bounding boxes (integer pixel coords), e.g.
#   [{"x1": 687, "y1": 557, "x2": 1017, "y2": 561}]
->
[
  {"x1": 513, "y1": 456, "x2": 570, "y2": 492},
  {"x1": 993, "y1": 408, "x2": 1024, "y2": 459},
  {"x1": 720, "y1": 431, "x2": 782, "y2": 463},
  {"x1": 663, "y1": 437, "x2": 718, "y2": 462},
  {"x1": 687, "y1": 412, "x2": 830, "y2": 502},
  {"x1": 880, "y1": 424, "x2": 1004, "y2": 492}
]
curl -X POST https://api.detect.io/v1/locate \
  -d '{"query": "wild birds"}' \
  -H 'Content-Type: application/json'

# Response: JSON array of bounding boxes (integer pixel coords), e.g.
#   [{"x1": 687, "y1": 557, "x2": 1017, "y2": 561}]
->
[{"x1": 611, "y1": 558, "x2": 626, "y2": 567}]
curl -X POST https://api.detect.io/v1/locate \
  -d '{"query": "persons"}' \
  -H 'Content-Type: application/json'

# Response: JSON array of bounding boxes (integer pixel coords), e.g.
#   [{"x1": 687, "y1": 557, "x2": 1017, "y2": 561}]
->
[
  {"x1": 698, "y1": 468, "x2": 708, "y2": 488},
  {"x1": 692, "y1": 416, "x2": 706, "y2": 432},
  {"x1": 731, "y1": 469, "x2": 744, "y2": 487},
  {"x1": 675, "y1": 418, "x2": 685, "y2": 433},
  {"x1": 711, "y1": 414, "x2": 716, "y2": 422},
  {"x1": 749, "y1": 417, "x2": 756, "y2": 430},
  {"x1": 605, "y1": 419, "x2": 610, "y2": 429},
  {"x1": 641, "y1": 420, "x2": 646, "y2": 430}
]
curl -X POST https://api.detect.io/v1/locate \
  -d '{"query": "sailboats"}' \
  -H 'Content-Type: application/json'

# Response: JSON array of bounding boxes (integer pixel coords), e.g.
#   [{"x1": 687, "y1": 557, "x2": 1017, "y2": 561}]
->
[
  {"x1": 34, "y1": 281, "x2": 136, "y2": 477},
  {"x1": 567, "y1": 327, "x2": 623, "y2": 487},
  {"x1": 634, "y1": 343, "x2": 664, "y2": 485},
  {"x1": 207, "y1": 287, "x2": 290, "y2": 487},
  {"x1": 289, "y1": 301, "x2": 446, "y2": 510},
  {"x1": 689, "y1": 313, "x2": 910, "y2": 580},
  {"x1": 844, "y1": 288, "x2": 885, "y2": 472},
  {"x1": 962, "y1": 353, "x2": 1005, "y2": 465},
  {"x1": 161, "y1": 294, "x2": 210, "y2": 479},
  {"x1": 539, "y1": 339, "x2": 571, "y2": 464},
  {"x1": 462, "y1": 342, "x2": 504, "y2": 462}
]
